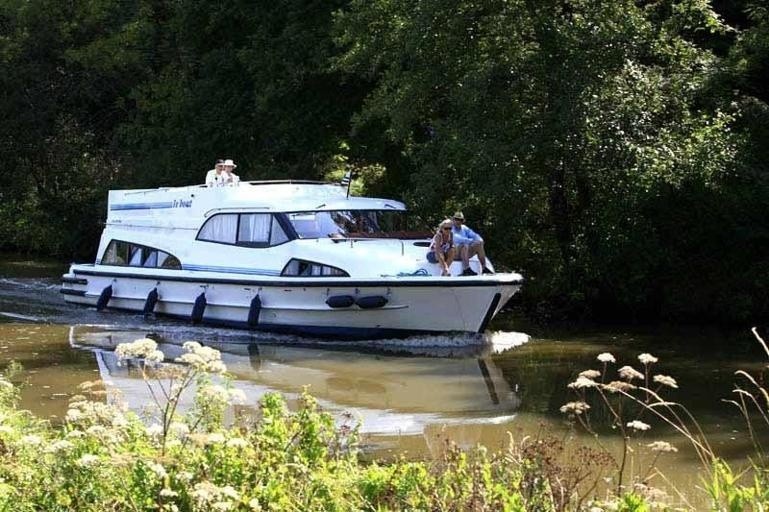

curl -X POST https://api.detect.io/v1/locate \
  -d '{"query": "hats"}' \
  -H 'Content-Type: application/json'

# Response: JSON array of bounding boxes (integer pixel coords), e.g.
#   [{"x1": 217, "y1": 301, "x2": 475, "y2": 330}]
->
[
  {"x1": 215, "y1": 159, "x2": 224, "y2": 165},
  {"x1": 225, "y1": 159, "x2": 237, "y2": 167},
  {"x1": 453, "y1": 212, "x2": 464, "y2": 219}
]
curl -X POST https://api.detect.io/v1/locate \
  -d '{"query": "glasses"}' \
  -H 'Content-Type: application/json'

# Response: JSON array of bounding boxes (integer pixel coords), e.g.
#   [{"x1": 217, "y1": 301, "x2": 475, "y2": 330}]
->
[
  {"x1": 444, "y1": 227, "x2": 452, "y2": 231},
  {"x1": 455, "y1": 217, "x2": 463, "y2": 221}
]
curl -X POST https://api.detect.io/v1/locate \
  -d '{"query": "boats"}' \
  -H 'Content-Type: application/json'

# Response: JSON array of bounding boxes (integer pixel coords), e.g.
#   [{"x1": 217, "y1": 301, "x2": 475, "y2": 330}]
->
[{"x1": 58, "y1": 174, "x2": 523, "y2": 341}]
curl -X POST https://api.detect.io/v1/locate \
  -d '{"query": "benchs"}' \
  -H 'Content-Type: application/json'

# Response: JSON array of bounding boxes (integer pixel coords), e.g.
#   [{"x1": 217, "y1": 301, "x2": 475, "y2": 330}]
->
[{"x1": 428, "y1": 260, "x2": 481, "y2": 275}]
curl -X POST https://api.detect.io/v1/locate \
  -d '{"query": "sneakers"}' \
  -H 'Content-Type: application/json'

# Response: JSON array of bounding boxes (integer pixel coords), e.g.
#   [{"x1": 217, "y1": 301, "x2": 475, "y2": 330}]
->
[
  {"x1": 482, "y1": 267, "x2": 493, "y2": 273},
  {"x1": 440, "y1": 268, "x2": 451, "y2": 276},
  {"x1": 462, "y1": 266, "x2": 477, "y2": 276}
]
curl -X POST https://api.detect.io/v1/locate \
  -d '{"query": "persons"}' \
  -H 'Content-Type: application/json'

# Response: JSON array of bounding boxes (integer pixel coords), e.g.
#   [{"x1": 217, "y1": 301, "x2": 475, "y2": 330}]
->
[
  {"x1": 426, "y1": 219, "x2": 456, "y2": 275},
  {"x1": 206, "y1": 159, "x2": 240, "y2": 187},
  {"x1": 450, "y1": 211, "x2": 494, "y2": 275}
]
